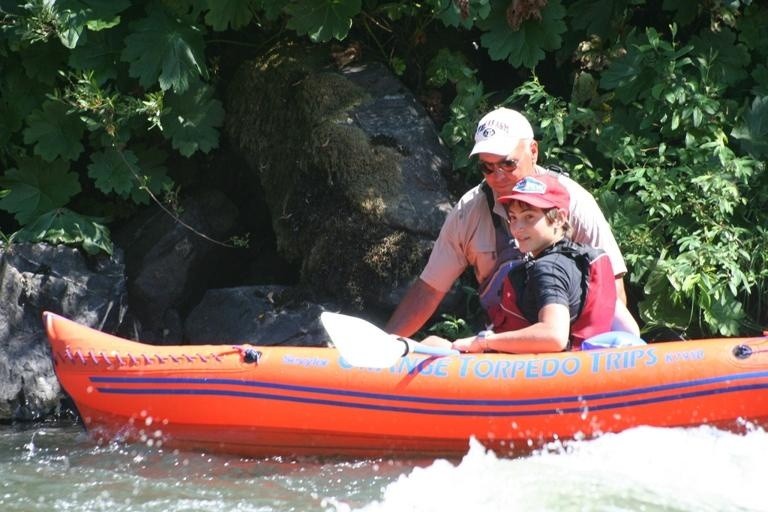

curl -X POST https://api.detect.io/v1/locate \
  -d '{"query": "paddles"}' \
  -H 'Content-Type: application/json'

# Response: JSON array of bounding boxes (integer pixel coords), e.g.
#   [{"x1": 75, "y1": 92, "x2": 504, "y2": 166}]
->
[{"x1": 321, "y1": 312, "x2": 461, "y2": 370}]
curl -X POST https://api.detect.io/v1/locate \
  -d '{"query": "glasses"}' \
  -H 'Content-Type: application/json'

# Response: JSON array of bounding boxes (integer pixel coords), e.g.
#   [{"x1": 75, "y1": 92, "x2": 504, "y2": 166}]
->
[{"x1": 477, "y1": 149, "x2": 526, "y2": 175}]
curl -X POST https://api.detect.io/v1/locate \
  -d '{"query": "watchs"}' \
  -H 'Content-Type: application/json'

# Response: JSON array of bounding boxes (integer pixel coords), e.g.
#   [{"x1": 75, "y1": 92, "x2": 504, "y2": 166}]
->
[{"x1": 477, "y1": 329, "x2": 494, "y2": 353}]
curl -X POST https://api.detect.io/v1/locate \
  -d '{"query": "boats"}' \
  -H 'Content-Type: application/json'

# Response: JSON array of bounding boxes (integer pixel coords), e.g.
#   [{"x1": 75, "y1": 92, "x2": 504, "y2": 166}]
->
[{"x1": 42, "y1": 311, "x2": 768, "y2": 457}]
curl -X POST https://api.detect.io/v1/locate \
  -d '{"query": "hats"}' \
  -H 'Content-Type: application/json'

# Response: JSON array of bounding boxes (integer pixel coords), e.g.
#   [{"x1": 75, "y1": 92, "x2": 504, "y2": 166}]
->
[
  {"x1": 496, "y1": 176, "x2": 571, "y2": 216},
  {"x1": 469, "y1": 106, "x2": 534, "y2": 159}
]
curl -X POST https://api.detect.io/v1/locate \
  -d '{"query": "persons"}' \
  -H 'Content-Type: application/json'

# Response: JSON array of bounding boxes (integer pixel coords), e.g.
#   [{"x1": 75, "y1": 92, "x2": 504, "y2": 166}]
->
[
  {"x1": 384, "y1": 107, "x2": 640, "y2": 349},
  {"x1": 451, "y1": 175, "x2": 616, "y2": 354}
]
